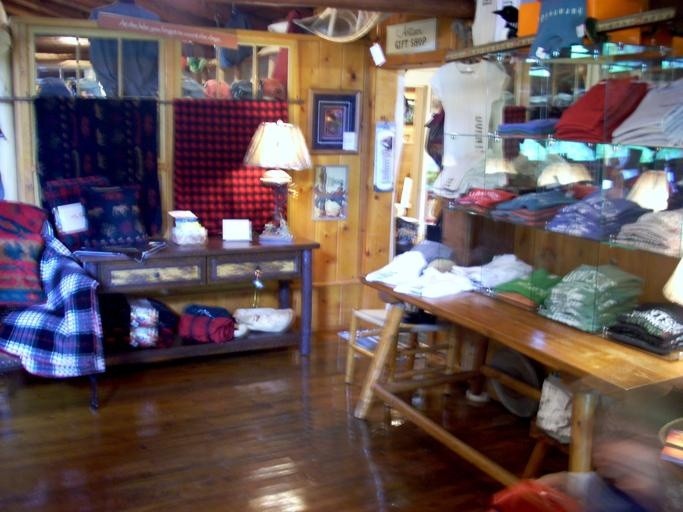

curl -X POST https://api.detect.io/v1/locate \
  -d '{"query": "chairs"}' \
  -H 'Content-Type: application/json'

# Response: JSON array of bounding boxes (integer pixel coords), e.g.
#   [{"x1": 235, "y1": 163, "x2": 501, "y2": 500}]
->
[{"x1": 0, "y1": 198, "x2": 100, "y2": 416}]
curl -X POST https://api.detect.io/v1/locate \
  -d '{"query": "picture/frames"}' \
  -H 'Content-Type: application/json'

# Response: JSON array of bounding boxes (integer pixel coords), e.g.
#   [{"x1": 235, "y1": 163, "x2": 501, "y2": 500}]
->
[
  {"x1": 311, "y1": 164, "x2": 349, "y2": 222},
  {"x1": 303, "y1": 86, "x2": 363, "y2": 158}
]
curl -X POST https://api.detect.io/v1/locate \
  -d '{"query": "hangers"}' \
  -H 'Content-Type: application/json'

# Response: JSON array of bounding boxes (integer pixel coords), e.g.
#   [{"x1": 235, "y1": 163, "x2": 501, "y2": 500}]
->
[{"x1": 456, "y1": 27, "x2": 488, "y2": 64}]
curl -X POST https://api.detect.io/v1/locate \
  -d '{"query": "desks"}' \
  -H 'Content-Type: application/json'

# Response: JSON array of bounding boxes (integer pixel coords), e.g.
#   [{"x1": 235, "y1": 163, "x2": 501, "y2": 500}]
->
[{"x1": 349, "y1": 263, "x2": 682, "y2": 490}]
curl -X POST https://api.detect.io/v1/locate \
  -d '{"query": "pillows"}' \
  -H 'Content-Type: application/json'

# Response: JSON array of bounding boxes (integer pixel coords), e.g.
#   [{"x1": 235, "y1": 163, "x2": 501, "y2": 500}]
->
[
  {"x1": 72, "y1": 180, "x2": 150, "y2": 249},
  {"x1": 0, "y1": 200, "x2": 48, "y2": 240},
  {"x1": 0, "y1": 232, "x2": 47, "y2": 312}
]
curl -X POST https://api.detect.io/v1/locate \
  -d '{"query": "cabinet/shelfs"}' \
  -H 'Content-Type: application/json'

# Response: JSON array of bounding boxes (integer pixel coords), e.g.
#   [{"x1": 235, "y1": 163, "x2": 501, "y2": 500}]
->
[
  {"x1": 66, "y1": 233, "x2": 320, "y2": 369},
  {"x1": 440, "y1": 8, "x2": 682, "y2": 356}
]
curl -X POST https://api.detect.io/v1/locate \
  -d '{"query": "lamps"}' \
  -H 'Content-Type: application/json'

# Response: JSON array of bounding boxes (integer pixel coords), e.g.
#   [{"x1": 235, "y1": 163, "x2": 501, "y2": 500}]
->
[{"x1": 238, "y1": 117, "x2": 313, "y2": 246}]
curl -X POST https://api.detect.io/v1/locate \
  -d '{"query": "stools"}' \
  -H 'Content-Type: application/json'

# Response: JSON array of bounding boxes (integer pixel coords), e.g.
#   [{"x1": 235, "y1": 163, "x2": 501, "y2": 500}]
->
[{"x1": 343, "y1": 304, "x2": 459, "y2": 420}]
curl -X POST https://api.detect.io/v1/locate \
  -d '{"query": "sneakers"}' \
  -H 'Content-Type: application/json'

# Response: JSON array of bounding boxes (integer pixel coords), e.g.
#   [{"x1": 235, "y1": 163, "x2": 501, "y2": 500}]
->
[{"x1": 234, "y1": 307, "x2": 297, "y2": 335}]
